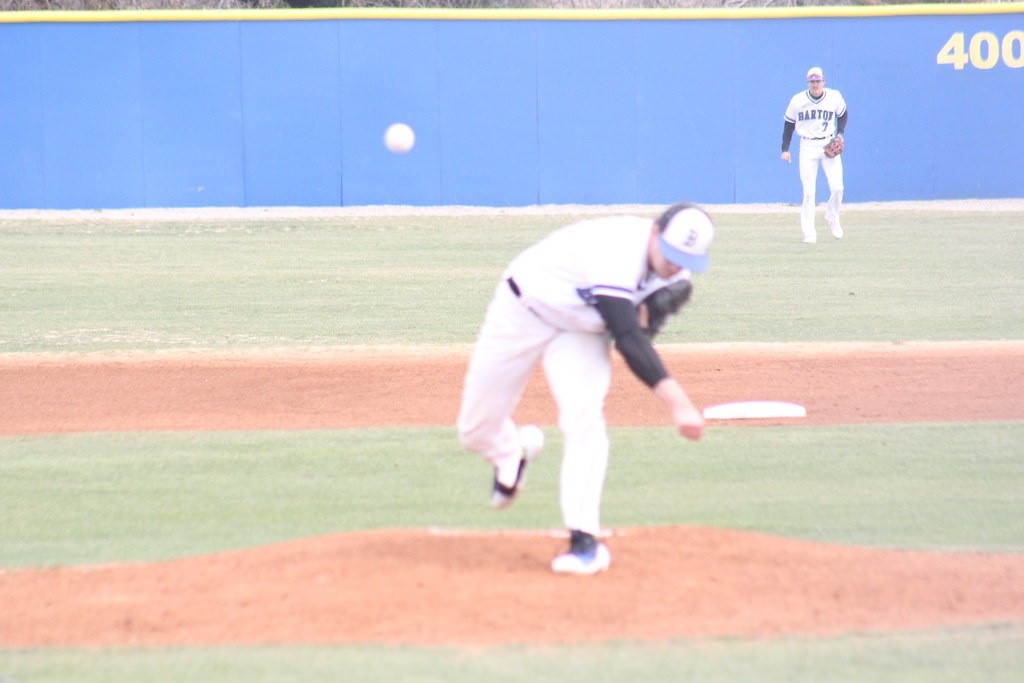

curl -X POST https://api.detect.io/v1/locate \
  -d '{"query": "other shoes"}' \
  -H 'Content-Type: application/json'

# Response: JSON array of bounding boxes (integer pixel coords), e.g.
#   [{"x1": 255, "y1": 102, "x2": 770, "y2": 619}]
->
[
  {"x1": 826, "y1": 215, "x2": 843, "y2": 239},
  {"x1": 493, "y1": 425, "x2": 544, "y2": 508},
  {"x1": 551, "y1": 541, "x2": 610, "y2": 575},
  {"x1": 803, "y1": 236, "x2": 816, "y2": 243}
]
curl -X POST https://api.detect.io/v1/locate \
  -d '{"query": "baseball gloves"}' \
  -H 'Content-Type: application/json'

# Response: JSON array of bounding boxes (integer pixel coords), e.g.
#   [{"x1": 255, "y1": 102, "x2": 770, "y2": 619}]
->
[
  {"x1": 824, "y1": 136, "x2": 844, "y2": 158},
  {"x1": 640, "y1": 279, "x2": 693, "y2": 338}
]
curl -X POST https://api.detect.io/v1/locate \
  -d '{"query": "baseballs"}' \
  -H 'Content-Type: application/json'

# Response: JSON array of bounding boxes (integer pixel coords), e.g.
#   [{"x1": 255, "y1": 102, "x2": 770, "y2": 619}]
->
[{"x1": 385, "y1": 122, "x2": 416, "y2": 154}]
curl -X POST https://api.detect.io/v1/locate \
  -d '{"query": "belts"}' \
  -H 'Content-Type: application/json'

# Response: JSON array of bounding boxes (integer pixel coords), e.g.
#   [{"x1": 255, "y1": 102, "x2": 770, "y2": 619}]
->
[
  {"x1": 507, "y1": 275, "x2": 541, "y2": 318},
  {"x1": 802, "y1": 134, "x2": 832, "y2": 141}
]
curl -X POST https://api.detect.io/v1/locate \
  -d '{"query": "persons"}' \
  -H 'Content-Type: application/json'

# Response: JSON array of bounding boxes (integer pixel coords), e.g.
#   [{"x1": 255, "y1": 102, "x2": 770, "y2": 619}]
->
[
  {"x1": 781, "y1": 66, "x2": 848, "y2": 244},
  {"x1": 457, "y1": 202, "x2": 716, "y2": 574}
]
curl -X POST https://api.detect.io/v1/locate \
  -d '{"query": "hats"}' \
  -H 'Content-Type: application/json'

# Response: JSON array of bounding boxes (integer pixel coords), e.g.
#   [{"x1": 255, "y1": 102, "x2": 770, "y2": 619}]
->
[
  {"x1": 657, "y1": 204, "x2": 714, "y2": 272},
  {"x1": 807, "y1": 67, "x2": 823, "y2": 82}
]
